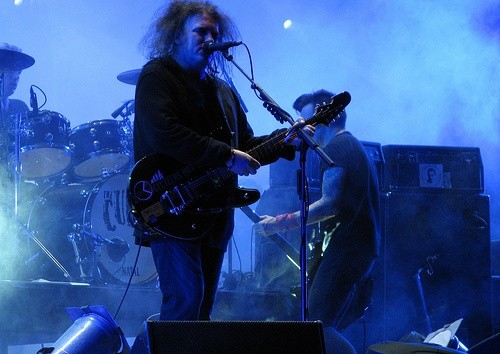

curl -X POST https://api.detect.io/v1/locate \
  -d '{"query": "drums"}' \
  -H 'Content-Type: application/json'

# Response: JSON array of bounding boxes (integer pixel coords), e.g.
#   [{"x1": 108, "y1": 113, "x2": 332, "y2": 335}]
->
[
  {"x1": 69, "y1": 119, "x2": 130, "y2": 178},
  {"x1": 6, "y1": 110, "x2": 75, "y2": 179},
  {"x1": 22, "y1": 172, "x2": 158, "y2": 286}
]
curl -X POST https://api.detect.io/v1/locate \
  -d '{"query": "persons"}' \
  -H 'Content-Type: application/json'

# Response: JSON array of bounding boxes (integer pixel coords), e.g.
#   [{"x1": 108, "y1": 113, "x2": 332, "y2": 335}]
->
[
  {"x1": 0, "y1": 41, "x2": 30, "y2": 158},
  {"x1": 252, "y1": 90, "x2": 382, "y2": 354},
  {"x1": 130, "y1": 0, "x2": 315, "y2": 354},
  {"x1": 426, "y1": 168, "x2": 436, "y2": 183}
]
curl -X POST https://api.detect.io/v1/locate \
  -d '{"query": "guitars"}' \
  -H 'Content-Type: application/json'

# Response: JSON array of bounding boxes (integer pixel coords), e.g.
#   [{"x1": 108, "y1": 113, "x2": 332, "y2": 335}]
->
[
  {"x1": 127, "y1": 92, "x2": 351, "y2": 244},
  {"x1": 239, "y1": 205, "x2": 372, "y2": 332}
]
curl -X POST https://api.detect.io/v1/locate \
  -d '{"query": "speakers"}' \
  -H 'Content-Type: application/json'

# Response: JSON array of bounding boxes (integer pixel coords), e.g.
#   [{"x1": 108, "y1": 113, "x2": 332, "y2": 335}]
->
[
  {"x1": 131, "y1": 320, "x2": 326, "y2": 354},
  {"x1": 255, "y1": 189, "x2": 494, "y2": 354}
]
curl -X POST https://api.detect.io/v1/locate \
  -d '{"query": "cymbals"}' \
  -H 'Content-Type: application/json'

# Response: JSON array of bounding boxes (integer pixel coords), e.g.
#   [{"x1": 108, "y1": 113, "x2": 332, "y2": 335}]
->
[{"x1": 117, "y1": 69, "x2": 144, "y2": 86}]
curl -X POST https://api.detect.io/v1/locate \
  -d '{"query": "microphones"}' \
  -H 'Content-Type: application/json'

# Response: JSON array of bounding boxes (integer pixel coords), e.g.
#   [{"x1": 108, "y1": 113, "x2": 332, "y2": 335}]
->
[
  {"x1": 203, "y1": 41, "x2": 242, "y2": 54},
  {"x1": 118, "y1": 242, "x2": 130, "y2": 254},
  {"x1": 111, "y1": 100, "x2": 131, "y2": 119},
  {"x1": 30, "y1": 87, "x2": 35, "y2": 107}
]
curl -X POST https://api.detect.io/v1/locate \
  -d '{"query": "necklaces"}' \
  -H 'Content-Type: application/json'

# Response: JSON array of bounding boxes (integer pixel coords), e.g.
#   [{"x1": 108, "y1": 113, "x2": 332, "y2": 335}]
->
[{"x1": 334, "y1": 126, "x2": 343, "y2": 135}]
